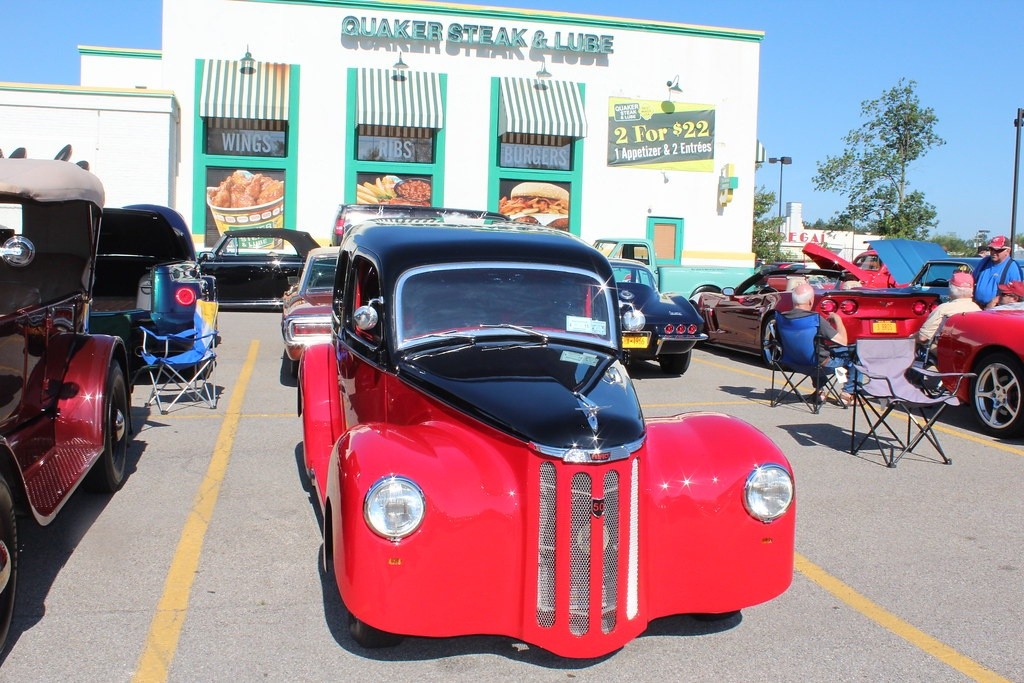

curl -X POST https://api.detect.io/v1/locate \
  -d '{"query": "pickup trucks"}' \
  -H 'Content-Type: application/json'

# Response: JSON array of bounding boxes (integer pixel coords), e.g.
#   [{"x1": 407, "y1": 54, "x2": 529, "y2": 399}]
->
[{"x1": 591, "y1": 237, "x2": 755, "y2": 295}]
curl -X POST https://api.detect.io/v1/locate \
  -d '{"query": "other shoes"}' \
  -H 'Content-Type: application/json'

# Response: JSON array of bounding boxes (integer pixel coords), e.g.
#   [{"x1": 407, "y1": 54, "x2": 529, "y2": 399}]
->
[
  {"x1": 930, "y1": 386, "x2": 940, "y2": 396},
  {"x1": 938, "y1": 383, "x2": 950, "y2": 394}
]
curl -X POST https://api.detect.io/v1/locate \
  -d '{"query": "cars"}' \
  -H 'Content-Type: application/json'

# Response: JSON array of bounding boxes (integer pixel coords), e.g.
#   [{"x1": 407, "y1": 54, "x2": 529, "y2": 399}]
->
[
  {"x1": 297, "y1": 216, "x2": 795, "y2": 657},
  {"x1": 870, "y1": 258, "x2": 1024, "y2": 302},
  {"x1": 696, "y1": 265, "x2": 944, "y2": 370},
  {"x1": 803, "y1": 241, "x2": 909, "y2": 292},
  {"x1": 605, "y1": 258, "x2": 707, "y2": 373},
  {"x1": 77, "y1": 205, "x2": 219, "y2": 377},
  {"x1": 934, "y1": 302, "x2": 1024, "y2": 439},
  {"x1": 194, "y1": 228, "x2": 332, "y2": 312},
  {"x1": 284, "y1": 247, "x2": 352, "y2": 375},
  {"x1": 0, "y1": 158, "x2": 130, "y2": 665}
]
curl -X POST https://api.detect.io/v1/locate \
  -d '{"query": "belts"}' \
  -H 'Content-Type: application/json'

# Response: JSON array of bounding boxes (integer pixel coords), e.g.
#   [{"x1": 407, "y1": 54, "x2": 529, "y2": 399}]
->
[{"x1": 819, "y1": 356, "x2": 830, "y2": 367}]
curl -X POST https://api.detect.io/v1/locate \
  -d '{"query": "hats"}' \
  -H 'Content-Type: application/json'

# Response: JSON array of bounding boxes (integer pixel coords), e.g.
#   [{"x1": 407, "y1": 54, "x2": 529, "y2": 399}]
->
[
  {"x1": 988, "y1": 235, "x2": 1011, "y2": 250},
  {"x1": 998, "y1": 281, "x2": 1024, "y2": 297},
  {"x1": 951, "y1": 272, "x2": 973, "y2": 288}
]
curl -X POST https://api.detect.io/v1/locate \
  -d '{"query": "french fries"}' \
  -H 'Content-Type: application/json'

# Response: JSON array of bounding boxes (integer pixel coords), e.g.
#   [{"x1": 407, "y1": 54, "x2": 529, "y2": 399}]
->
[{"x1": 356, "y1": 178, "x2": 395, "y2": 204}]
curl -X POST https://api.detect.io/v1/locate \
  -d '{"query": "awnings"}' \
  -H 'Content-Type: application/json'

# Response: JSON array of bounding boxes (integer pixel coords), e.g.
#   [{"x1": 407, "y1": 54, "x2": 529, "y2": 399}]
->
[
  {"x1": 198, "y1": 59, "x2": 290, "y2": 121},
  {"x1": 498, "y1": 77, "x2": 587, "y2": 142},
  {"x1": 354, "y1": 67, "x2": 445, "y2": 137}
]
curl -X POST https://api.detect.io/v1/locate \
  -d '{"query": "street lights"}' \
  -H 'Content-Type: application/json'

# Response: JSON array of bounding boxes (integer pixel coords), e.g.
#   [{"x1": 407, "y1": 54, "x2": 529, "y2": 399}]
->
[{"x1": 769, "y1": 156, "x2": 792, "y2": 246}]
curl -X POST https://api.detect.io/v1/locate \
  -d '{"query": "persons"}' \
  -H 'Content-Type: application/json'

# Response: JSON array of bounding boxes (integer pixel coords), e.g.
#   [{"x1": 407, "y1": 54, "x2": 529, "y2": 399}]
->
[
  {"x1": 918, "y1": 272, "x2": 983, "y2": 358},
  {"x1": 998, "y1": 281, "x2": 1024, "y2": 306},
  {"x1": 972, "y1": 235, "x2": 1023, "y2": 310},
  {"x1": 780, "y1": 283, "x2": 867, "y2": 406},
  {"x1": 973, "y1": 246, "x2": 989, "y2": 258}
]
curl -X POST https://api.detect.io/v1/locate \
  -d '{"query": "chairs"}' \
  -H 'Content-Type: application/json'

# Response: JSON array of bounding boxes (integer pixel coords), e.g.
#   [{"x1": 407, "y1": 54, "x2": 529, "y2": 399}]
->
[
  {"x1": 759, "y1": 308, "x2": 856, "y2": 414},
  {"x1": 129, "y1": 297, "x2": 219, "y2": 414},
  {"x1": 914, "y1": 313, "x2": 955, "y2": 394},
  {"x1": 850, "y1": 338, "x2": 978, "y2": 468}
]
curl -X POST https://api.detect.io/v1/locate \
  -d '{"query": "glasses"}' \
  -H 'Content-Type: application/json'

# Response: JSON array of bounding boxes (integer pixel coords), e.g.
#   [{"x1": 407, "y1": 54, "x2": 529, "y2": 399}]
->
[
  {"x1": 1001, "y1": 292, "x2": 1015, "y2": 297},
  {"x1": 989, "y1": 247, "x2": 1006, "y2": 253}
]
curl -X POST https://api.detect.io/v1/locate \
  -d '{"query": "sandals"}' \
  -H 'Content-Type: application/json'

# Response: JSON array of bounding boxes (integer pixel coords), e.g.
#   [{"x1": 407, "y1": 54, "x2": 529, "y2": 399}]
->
[
  {"x1": 837, "y1": 394, "x2": 867, "y2": 405},
  {"x1": 813, "y1": 389, "x2": 826, "y2": 404}
]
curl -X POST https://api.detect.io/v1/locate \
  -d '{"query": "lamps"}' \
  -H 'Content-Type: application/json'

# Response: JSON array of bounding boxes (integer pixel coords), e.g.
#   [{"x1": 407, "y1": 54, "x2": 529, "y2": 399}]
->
[
  {"x1": 667, "y1": 73, "x2": 683, "y2": 94},
  {"x1": 394, "y1": 51, "x2": 409, "y2": 68},
  {"x1": 535, "y1": 60, "x2": 551, "y2": 78},
  {"x1": 241, "y1": 45, "x2": 256, "y2": 62}
]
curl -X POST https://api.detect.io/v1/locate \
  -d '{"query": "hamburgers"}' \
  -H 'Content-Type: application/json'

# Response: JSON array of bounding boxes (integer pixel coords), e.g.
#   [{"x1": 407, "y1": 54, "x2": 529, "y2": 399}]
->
[{"x1": 511, "y1": 182, "x2": 569, "y2": 230}]
